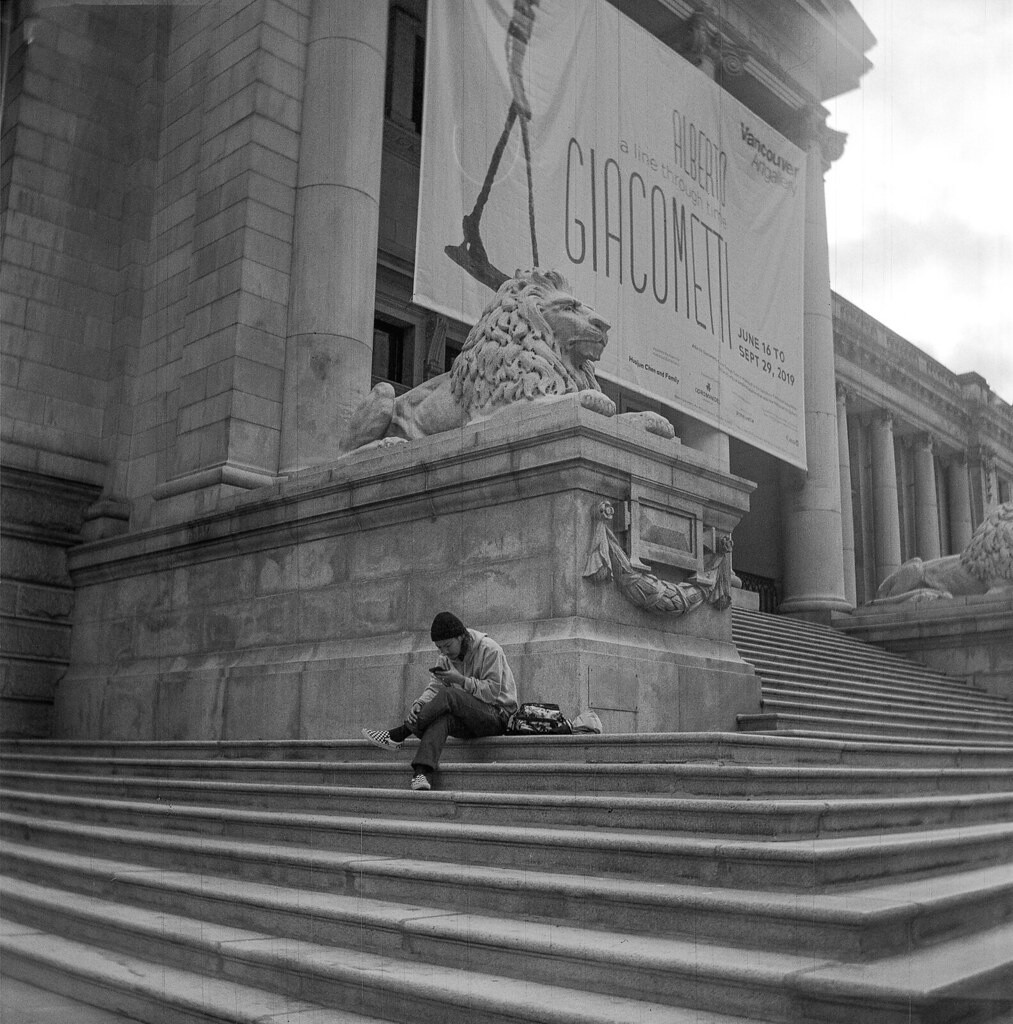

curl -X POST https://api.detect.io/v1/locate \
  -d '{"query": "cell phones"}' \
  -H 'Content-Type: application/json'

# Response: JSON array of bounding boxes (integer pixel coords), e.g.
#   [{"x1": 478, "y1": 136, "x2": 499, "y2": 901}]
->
[{"x1": 429, "y1": 666, "x2": 445, "y2": 674}]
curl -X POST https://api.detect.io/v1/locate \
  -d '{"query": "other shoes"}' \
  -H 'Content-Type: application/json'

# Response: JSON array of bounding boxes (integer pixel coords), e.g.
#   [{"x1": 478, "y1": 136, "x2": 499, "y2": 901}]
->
[
  {"x1": 361, "y1": 728, "x2": 403, "y2": 751},
  {"x1": 411, "y1": 774, "x2": 431, "y2": 790}
]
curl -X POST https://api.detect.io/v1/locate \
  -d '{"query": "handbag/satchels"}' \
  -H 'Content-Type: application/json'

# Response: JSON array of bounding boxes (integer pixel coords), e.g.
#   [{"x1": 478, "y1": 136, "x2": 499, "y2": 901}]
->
[{"x1": 505, "y1": 703, "x2": 573, "y2": 735}]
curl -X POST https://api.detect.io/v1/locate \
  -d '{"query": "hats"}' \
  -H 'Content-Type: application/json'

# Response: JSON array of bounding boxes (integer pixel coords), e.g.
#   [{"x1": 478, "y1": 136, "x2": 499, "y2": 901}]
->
[{"x1": 430, "y1": 612, "x2": 466, "y2": 642}]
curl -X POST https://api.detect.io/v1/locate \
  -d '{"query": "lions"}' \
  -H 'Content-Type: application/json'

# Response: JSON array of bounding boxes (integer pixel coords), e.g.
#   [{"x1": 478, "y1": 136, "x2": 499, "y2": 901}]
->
[
  {"x1": 335, "y1": 266, "x2": 675, "y2": 439},
  {"x1": 864, "y1": 500, "x2": 1012, "y2": 606}
]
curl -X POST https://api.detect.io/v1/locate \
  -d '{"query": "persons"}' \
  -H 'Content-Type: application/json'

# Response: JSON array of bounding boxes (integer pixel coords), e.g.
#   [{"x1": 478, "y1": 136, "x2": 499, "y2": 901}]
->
[{"x1": 361, "y1": 611, "x2": 518, "y2": 790}]
